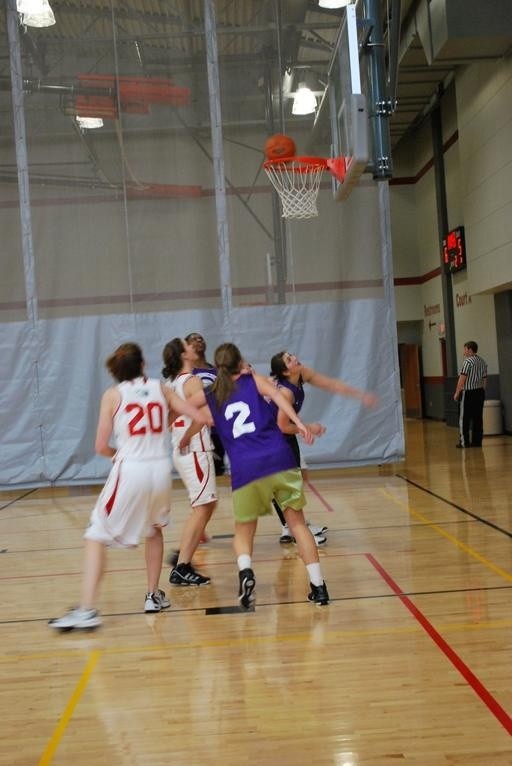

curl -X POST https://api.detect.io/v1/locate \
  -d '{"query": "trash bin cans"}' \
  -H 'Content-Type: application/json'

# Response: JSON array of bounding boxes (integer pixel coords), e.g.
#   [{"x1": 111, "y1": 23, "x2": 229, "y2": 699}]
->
[{"x1": 483, "y1": 400, "x2": 503, "y2": 437}]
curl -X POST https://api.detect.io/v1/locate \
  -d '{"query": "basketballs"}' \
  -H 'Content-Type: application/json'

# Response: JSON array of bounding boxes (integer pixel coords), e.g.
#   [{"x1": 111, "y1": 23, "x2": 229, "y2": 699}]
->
[{"x1": 264, "y1": 134, "x2": 296, "y2": 164}]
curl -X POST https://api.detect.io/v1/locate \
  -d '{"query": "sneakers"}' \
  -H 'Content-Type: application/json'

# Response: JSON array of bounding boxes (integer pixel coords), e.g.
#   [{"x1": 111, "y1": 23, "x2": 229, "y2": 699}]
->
[
  {"x1": 144, "y1": 589, "x2": 170, "y2": 610},
  {"x1": 169, "y1": 562, "x2": 210, "y2": 585},
  {"x1": 47, "y1": 607, "x2": 102, "y2": 627},
  {"x1": 239, "y1": 568, "x2": 256, "y2": 612},
  {"x1": 280, "y1": 519, "x2": 329, "y2": 546},
  {"x1": 306, "y1": 579, "x2": 329, "y2": 606}
]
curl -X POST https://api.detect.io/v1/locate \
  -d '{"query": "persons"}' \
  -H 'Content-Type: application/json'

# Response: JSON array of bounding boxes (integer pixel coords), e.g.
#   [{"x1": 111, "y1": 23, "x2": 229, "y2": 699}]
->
[
  {"x1": 454, "y1": 341, "x2": 488, "y2": 448},
  {"x1": 46, "y1": 341, "x2": 209, "y2": 628},
  {"x1": 161, "y1": 334, "x2": 376, "y2": 612}
]
